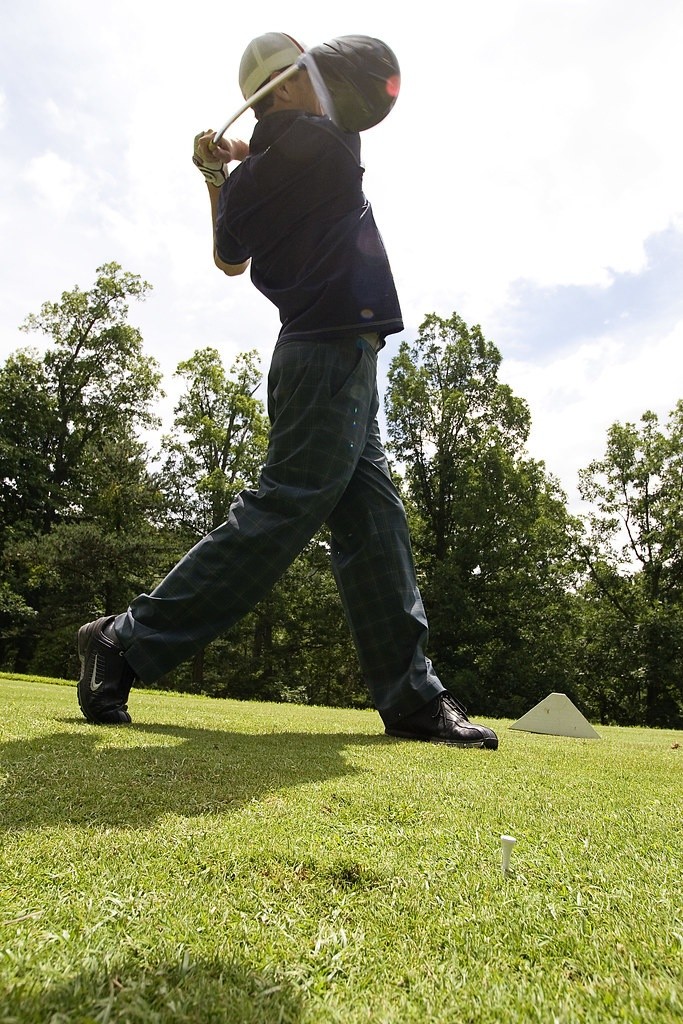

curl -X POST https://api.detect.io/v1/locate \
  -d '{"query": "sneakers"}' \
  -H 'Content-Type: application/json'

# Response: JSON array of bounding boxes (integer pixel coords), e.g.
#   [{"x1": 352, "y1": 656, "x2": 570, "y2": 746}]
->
[
  {"x1": 76, "y1": 617, "x2": 136, "y2": 726},
  {"x1": 384, "y1": 688, "x2": 497, "y2": 751}
]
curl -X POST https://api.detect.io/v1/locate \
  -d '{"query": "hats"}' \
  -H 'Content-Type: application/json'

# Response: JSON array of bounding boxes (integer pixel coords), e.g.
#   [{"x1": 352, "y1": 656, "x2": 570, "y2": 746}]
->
[{"x1": 239, "y1": 32, "x2": 305, "y2": 102}]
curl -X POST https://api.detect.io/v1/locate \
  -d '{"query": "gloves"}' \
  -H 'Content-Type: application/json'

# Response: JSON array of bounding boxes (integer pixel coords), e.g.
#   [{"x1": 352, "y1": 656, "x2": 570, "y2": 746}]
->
[{"x1": 191, "y1": 131, "x2": 230, "y2": 189}]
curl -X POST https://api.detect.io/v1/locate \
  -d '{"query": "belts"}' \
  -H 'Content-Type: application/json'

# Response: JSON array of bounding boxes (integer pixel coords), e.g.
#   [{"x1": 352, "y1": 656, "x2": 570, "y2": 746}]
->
[{"x1": 360, "y1": 330, "x2": 382, "y2": 355}]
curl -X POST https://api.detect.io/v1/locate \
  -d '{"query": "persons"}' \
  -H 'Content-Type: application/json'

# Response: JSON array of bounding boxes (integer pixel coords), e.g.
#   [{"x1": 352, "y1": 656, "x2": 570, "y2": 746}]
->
[{"x1": 74, "y1": 30, "x2": 500, "y2": 750}]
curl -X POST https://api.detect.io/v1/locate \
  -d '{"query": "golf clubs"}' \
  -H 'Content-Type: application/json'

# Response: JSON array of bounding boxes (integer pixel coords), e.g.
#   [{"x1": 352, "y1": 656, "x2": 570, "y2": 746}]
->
[{"x1": 210, "y1": 34, "x2": 402, "y2": 133}]
